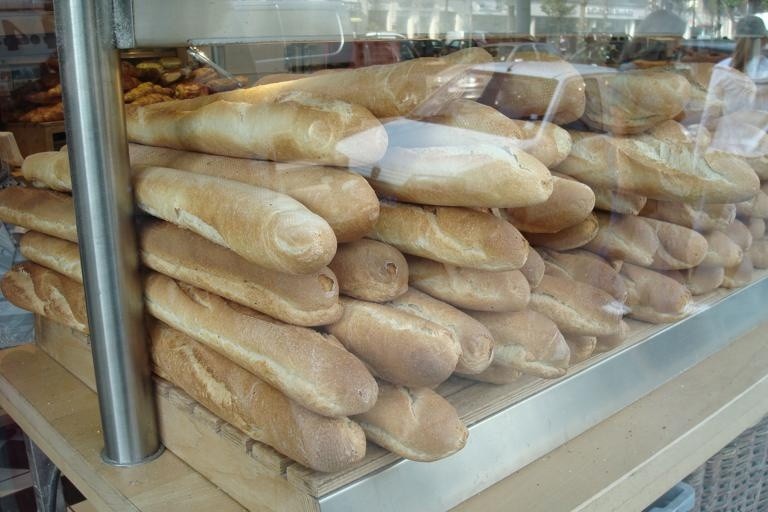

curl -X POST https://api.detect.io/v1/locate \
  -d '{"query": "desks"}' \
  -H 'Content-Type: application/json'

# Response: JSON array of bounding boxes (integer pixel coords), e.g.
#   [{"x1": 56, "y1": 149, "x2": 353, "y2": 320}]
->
[{"x1": 0, "y1": 119, "x2": 66, "y2": 161}]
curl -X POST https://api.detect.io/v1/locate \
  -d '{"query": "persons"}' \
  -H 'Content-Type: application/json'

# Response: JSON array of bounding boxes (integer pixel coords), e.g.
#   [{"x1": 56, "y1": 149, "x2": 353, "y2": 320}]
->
[{"x1": 590, "y1": 6, "x2": 768, "y2": 274}]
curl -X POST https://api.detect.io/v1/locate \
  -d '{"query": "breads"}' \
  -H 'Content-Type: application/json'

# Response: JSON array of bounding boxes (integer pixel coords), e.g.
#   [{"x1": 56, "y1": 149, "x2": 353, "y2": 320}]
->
[{"x1": 1, "y1": 47, "x2": 767, "y2": 475}]
